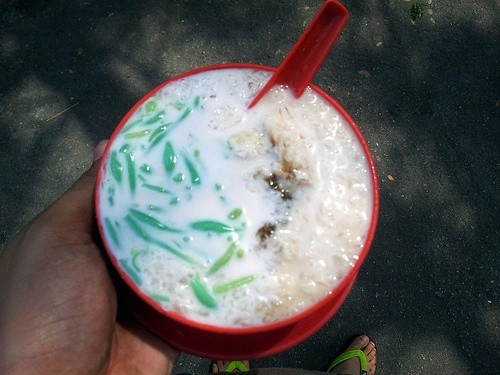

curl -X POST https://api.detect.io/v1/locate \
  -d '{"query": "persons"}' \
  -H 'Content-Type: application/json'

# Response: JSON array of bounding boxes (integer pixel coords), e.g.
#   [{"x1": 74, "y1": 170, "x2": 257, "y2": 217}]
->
[{"x1": 0, "y1": 138, "x2": 377, "y2": 374}]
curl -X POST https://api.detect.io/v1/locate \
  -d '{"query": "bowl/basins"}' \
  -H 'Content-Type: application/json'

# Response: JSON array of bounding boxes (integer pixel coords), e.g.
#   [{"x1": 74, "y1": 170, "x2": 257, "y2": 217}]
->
[{"x1": 90, "y1": 64, "x2": 380, "y2": 361}]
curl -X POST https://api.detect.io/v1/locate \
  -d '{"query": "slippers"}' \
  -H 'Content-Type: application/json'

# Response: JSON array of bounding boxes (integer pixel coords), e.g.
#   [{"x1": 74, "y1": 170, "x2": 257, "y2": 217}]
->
[
  {"x1": 326, "y1": 332, "x2": 379, "y2": 374},
  {"x1": 208, "y1": 358, "x2": 260, "y2": 375}
]
